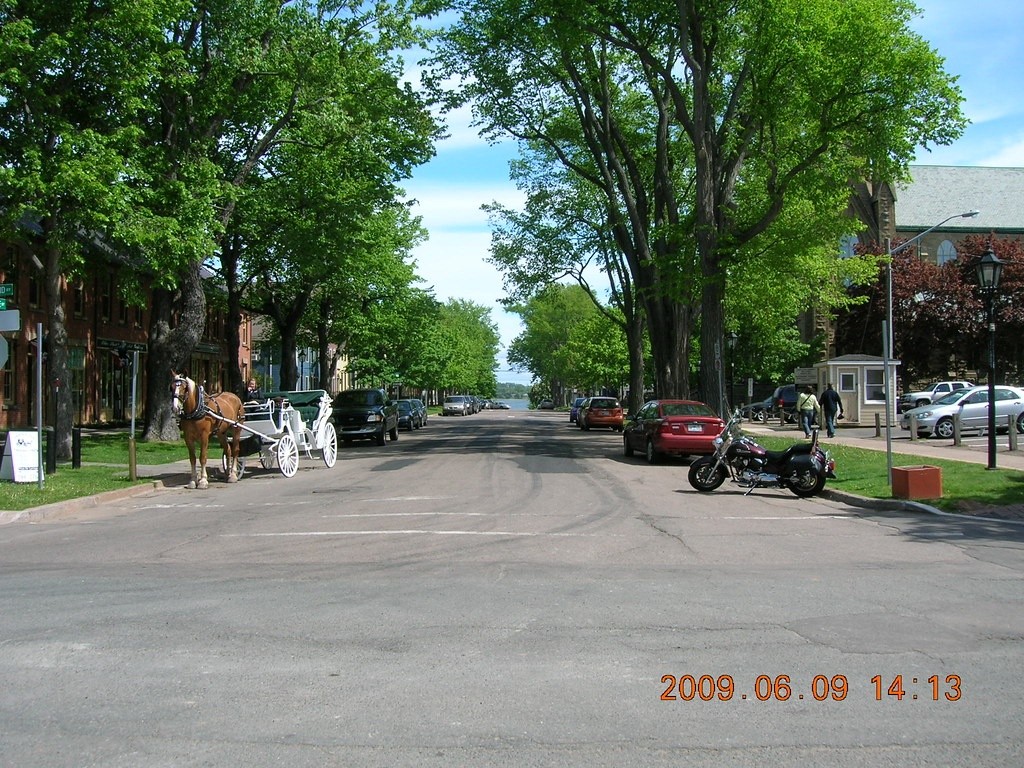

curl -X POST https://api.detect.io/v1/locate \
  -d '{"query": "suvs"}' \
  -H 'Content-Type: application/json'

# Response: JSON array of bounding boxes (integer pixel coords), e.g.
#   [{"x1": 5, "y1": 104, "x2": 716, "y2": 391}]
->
[
  {"x1": 897, "y1": 382, "x2": 976, "y2": 410},
  {"x1": 773, "y1": 385, "x2": 816, "y2": 424}
]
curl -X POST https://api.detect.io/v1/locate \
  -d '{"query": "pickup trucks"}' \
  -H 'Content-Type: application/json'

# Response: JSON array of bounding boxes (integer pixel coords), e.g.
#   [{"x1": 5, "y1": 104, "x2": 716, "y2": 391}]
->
[{"x1": 329, "y1": 388, "x2": 399, "y2": 448}]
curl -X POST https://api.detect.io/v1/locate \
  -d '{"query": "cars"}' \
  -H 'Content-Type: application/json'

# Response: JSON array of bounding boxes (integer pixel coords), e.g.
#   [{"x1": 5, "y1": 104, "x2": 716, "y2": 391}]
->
[
  {"x1": 395, "y1": 400, "x2": 419, "y2": 431},
  {"x1": 623, "y1": 399, "x2": 729, "y2": 466},
  {"x1": 740, "y1": 397, "x2": 780, "y2": 421},
  {"x1": 537, "y1": 399, "x2": 555, "y2": 409},
  {"x1": 442, "y1": 395, "x2": 469, "y2": 416},
  {"x1": 492, "y1": 401, "x2": 511, "y2": 410},
  {"x1": 570, "y1": 398, "x2": 586, "y2": 423},
  {"x1": 900, "y1": 385, "x2": 1024, "y2": 440},
  {"x1": 409, "y1": 398, "x2": 427, "y2": 427},
  {"x1": 463, "y1": 395, "x2": 495, "y2": 415},
  {"x1": 578, "y1": 397, "x2": 624, "y2": 432}
]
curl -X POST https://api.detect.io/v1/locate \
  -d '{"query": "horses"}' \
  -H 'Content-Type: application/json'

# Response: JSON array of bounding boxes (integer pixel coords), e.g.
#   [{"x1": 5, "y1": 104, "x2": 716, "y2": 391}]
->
[{"x1": 170, "y1": 368, "x2": 245, "y2": 489}]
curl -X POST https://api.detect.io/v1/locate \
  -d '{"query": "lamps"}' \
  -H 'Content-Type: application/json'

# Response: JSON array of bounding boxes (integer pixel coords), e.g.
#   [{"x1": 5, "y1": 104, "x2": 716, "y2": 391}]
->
[{"x1": 283, "y1": 402, "x2": 296, "y2": 423}]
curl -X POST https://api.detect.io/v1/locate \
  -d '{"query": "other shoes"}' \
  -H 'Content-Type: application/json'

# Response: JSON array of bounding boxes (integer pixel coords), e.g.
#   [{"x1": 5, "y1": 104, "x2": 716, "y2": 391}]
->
[
  {"x1": 829, "y1": 434, "x2": 836, "y2": 438},
  {"x1": 806, "y1": 433, "x2": 811, "y2": 439}
]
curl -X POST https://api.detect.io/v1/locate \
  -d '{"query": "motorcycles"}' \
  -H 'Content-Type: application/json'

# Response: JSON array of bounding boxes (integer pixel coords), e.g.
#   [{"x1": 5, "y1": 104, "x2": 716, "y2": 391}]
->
[{"x1": 687, "y1": 405, "x2": 837, "y2": 498}]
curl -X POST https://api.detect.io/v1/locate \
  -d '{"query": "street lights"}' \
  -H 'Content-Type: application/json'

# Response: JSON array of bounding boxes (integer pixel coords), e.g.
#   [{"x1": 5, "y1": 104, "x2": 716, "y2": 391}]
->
[
  {"x1": 881, "y1": 207, "x2": 980, "y2": 487},
  {"x1": 976, "y1": 238, "x2": 1003, "y2": 473}
]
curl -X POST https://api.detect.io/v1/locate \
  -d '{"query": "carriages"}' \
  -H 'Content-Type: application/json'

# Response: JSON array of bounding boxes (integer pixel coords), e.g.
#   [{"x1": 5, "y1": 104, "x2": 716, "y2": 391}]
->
[{"x1": 166, "y1": 368, "x2": 339, "y2": 492}]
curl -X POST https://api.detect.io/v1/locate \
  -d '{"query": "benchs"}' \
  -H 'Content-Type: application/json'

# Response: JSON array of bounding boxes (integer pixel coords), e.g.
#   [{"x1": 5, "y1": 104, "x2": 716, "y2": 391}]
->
[{"x1": 246, "y1": 391, "x2": 325, "y2": 431}]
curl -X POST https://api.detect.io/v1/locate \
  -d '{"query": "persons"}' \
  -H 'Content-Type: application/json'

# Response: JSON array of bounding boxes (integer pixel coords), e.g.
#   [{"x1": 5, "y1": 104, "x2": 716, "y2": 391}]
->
[
  {"x1": 242, "y1": 378, "x2": 266, "y2": 404},
  {"x1": 819, "y1": 382, "x2": 844, "y2": 438},
  {"x1": 797, "y1": 386, "x2": 820, "y2": 438}
]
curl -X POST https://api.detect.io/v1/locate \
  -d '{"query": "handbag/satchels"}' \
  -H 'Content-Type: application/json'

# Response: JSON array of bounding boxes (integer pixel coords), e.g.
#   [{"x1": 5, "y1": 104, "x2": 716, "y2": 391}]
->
[
  {"x1": 837, "y1": 414, "x2": 844, "y2": 420},
  {"x1": 789, "y1": 406, "x2": 796, "y2": 414}
]
